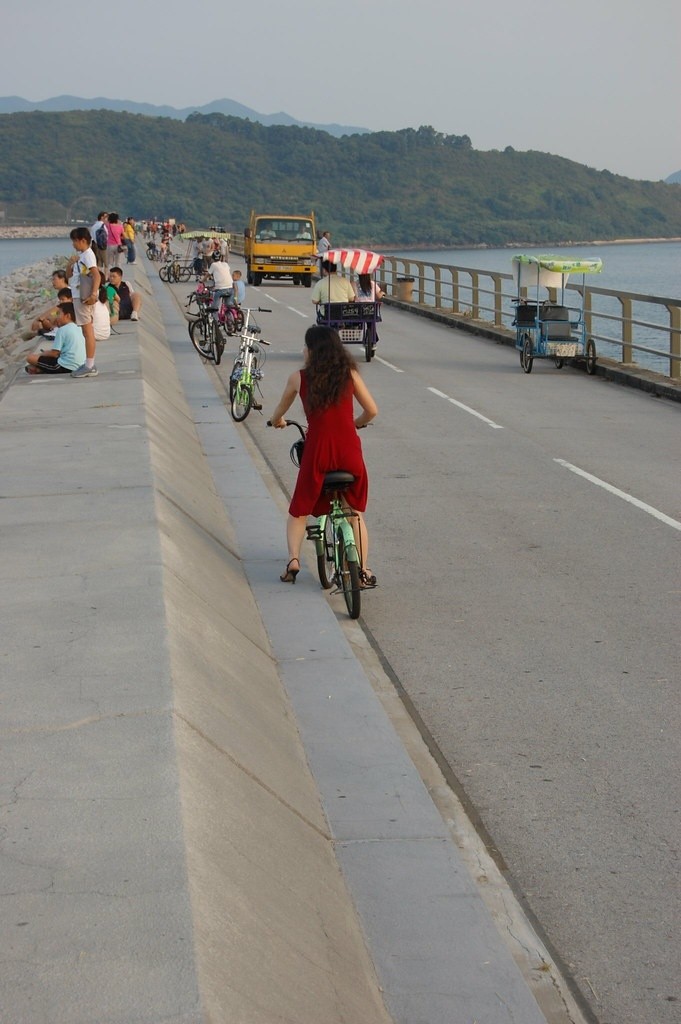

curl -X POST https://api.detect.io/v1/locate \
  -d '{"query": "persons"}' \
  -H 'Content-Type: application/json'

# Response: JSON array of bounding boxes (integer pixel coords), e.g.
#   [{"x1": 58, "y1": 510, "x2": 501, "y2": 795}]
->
[
  {"x1": 295, "y1": 224, "x2": 311, "y2": 239},
  {"x1": 260, "y1": 222, "x2": 277, "y2": 237},
  {"x1": 199, "y1": 250, "x2": 234, "y2": 327},
  {"x1": 90, "y1": 211, "x2": 137, "y2": 274},
  {"x1": 64, "y1": 228, "x2": 101, "y2": 377},
  {"x1": 317, "y1": 231, "x2": 332, "y2": 278},
  {"x1": 142, "y1": 221, "x2": 186, "y2": 261},
  {"x1": 312, "y1": 260, "x2": 355, "y2": 324},
  {"x1": 272, "y1": 325, "x2": 378, "y2": 585},
  {"x1": 231, "y1": 270, "x2": 246, "y2": 306},
  {"x1": 192, "y1": 226, "x2": 227, "y2": 281},
  {"x1": 350, "y1": 271, "x2": 383, "y2": 349},
  {"x1": 26, "y1": 267, "x2": 140, "y2": 374}
]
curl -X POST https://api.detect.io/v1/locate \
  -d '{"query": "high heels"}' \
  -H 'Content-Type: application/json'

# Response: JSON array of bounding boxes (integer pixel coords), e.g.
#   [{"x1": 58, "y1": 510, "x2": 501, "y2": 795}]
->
[
  {"x1": 360, "y1": 571, "x2": 377, "y2": 584},
  {"x1": 279, "y1": 558, "x2": 300, "y2": 584}
]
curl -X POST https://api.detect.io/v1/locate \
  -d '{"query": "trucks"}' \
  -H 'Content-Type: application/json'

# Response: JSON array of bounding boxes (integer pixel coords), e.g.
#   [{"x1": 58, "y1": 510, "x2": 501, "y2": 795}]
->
[{"x1": 244, "y1": 209, "x2": 322, "y2": 288}]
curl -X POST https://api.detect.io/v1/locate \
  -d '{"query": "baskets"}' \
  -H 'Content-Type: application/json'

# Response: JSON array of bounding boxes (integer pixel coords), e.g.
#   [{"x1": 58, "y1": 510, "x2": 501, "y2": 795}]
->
[{"x1": 293, "y1": 445, "x2": 304, "y2": 465}]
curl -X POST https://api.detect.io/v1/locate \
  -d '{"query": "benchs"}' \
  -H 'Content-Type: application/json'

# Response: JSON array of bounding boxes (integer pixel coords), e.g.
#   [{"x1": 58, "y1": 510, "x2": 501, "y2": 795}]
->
[
  {"x1": 515, "y1": 306, "x2": 582, "y2": 329},
  {"x1": 316, "y1": 302, "x2": 383, "y2": 322}
]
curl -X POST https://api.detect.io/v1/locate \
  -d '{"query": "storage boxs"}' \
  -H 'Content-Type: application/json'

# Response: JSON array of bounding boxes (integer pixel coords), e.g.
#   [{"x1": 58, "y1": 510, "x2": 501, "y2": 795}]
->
[{"x1": 541, "y1": 336, "x2": 578, "y2": 358}]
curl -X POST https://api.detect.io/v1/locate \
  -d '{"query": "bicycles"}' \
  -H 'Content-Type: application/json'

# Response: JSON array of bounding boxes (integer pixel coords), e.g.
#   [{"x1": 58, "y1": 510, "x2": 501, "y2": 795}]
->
[
  {"x1": 266, "y1": 418, "x2": 378, "y2": 620},
  {"x1": 184, "y1": 274, "x2": 272, "y2": 422},
  {"x1": 146, "y1": 238, "x2": 192, "y2": 285}
]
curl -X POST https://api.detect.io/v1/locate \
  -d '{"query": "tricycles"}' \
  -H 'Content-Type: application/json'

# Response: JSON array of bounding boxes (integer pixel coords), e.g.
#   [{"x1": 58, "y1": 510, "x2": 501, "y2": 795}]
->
[
  {"x1": 507, "y1": 253, "x2": 602, "y2": 377},
  {"x1": 311, "y1": 257, "x2": 384, "y2": 362}
]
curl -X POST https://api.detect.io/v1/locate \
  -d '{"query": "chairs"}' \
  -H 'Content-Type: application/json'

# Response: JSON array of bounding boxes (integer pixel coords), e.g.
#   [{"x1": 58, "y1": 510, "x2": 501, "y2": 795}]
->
[{"x1": 541, "y1": 305, "x2": 571, "y2": 336}]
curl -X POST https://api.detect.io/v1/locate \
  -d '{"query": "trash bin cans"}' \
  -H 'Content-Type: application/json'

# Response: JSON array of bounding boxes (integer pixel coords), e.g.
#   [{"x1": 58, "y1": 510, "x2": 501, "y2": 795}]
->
[{"x1": 397, "y1": 278, "x2": 415, "y2": 300}]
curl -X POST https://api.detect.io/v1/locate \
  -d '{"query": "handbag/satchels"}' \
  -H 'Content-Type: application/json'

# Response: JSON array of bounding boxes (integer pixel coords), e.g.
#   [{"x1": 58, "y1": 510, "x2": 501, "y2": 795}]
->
[
  {"x1": 80, "y1": 276, "x2": 99, "y2": 302},
  {"x1": 96, "y1": 224, "x2": 108, "y2": 250},
  {"x1": 118, "y1": 245, "x2": 124, "y2": 252}
]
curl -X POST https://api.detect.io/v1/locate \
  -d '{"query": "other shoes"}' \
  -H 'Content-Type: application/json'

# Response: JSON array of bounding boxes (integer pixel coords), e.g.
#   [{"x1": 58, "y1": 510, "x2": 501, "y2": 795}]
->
[
  {"x1": 24, "y1": 365, "x2": 43, "y2": 374},
  {"x1": 128, "y1": 261, "x2": 137, "y2": 265},
  {"x1": 44, "y1": 327, "x2": 58, "y2": 340},
  {"x1": 131, "y1": 312, "x2": 138, "y2": 321}
]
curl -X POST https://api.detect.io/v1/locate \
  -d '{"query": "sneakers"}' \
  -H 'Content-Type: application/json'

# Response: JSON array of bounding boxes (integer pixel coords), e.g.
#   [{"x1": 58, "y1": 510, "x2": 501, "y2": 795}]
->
[{"x1": 70, "y1": 364, "x2": 98, "y2": 378}]
